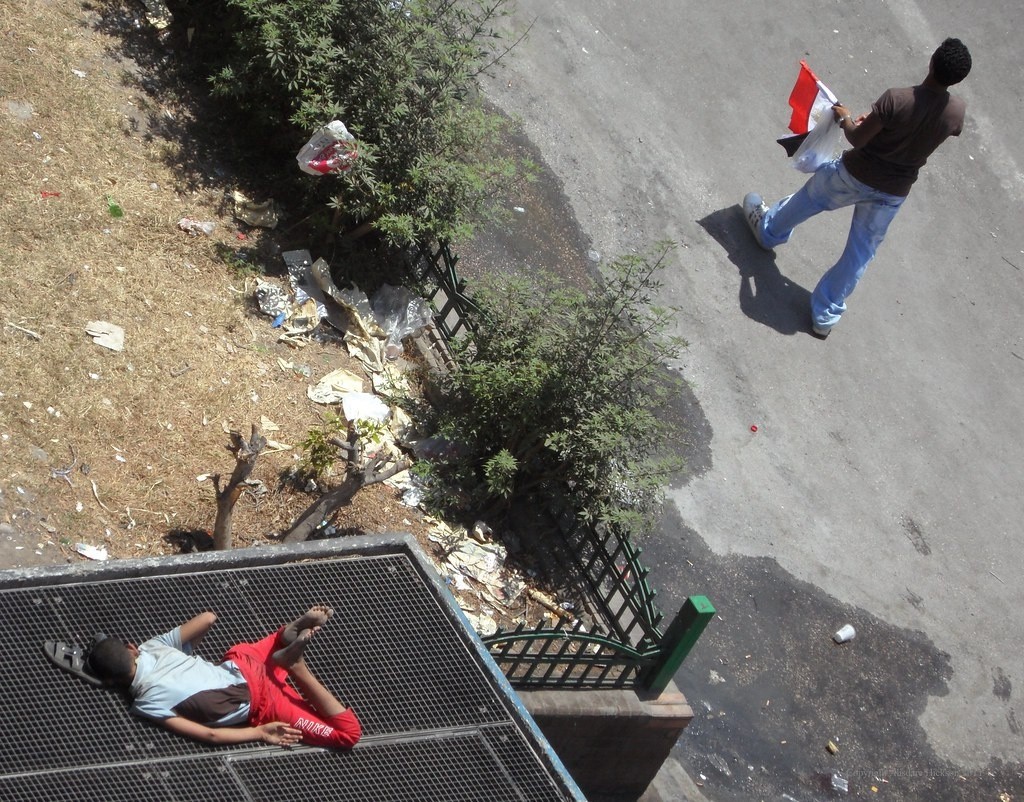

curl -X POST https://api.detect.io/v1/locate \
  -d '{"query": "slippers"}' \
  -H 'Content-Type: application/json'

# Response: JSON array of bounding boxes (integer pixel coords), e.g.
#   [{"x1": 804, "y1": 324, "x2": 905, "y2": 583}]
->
[{"x1": 45, "y1": 633, "x2": 108, "y2": 686}]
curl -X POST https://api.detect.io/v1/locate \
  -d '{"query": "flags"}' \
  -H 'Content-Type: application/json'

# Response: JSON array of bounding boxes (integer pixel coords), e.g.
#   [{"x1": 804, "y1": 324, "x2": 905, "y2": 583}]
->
[{"x1": 786, "y1": 59, "x2": 839, "y2": 134}]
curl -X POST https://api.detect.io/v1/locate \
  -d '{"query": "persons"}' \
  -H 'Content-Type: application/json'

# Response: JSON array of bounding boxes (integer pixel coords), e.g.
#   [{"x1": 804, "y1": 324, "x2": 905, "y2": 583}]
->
[
  {"x1": 742, "y1": 36, "x2": 973, "y2": 338},
  {"x1": 87, "y1": 604, "x2": 362, "y2": 749}
]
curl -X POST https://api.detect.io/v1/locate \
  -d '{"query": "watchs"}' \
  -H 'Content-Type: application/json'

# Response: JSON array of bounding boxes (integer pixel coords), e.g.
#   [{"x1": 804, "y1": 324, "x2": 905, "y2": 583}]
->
[{"x1": 839, "y1": 114, "x2": 853, "y2": 128}]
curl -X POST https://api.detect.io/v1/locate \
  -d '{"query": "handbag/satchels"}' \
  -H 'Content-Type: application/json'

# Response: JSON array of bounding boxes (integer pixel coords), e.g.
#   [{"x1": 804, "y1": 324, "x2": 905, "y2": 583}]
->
[{"x1": 777, "y1": 104, "x2": 851, "y2": 174}]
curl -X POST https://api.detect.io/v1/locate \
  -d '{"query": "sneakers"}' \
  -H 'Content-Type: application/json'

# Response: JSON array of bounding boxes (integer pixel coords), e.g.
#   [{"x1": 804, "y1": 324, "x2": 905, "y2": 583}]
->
[
  {"x1": 812, "y1": 312, "x2": 831, "y2": 336},
  {"x1": 743, "y1": 192, "x2": 770, "y2": 249}
]
